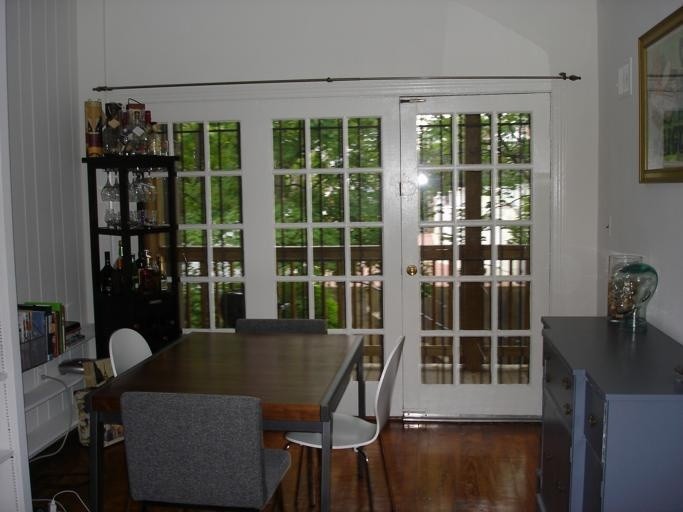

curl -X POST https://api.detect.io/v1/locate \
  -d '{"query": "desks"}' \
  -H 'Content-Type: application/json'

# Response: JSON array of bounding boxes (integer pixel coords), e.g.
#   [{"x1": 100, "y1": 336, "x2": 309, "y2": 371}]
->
[{"x1": 83, "y1": 331, "x2": 365, "y2": 512}]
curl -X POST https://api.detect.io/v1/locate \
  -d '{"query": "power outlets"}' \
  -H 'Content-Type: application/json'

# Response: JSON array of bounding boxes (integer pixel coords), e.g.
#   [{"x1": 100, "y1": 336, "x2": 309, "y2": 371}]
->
[{"x1": 34, "y1": 364, "x2": 48, "y2": 386}]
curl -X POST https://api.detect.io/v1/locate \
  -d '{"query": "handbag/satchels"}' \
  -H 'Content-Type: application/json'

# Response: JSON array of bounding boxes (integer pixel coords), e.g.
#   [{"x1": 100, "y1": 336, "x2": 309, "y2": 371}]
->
[{"x1": 73, "y1": 360, "x2": 125, "y2": 448}]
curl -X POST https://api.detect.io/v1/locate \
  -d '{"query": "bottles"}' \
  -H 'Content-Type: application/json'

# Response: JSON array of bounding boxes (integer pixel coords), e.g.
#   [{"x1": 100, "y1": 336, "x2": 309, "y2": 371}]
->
[{"x1": 99, "y1": 240, "x2": 168, "y2": 293}]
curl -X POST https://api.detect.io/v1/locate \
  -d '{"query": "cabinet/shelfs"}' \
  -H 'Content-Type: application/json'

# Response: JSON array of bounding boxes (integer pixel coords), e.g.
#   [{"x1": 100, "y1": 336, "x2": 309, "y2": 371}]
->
[
  {"x1": 23, "y1": 335, "x2": 90, "y2": 459},
  {"x1": 535, "y1": 316, "x2": 683, "y2": 512},
  {"x1": 81, "y1": 153, "x2": 183, "y2": 382}
]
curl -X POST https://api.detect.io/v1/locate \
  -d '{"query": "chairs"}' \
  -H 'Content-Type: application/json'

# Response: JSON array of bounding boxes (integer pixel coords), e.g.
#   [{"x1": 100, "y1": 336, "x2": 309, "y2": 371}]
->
[
  {"x1": 121, "y1": 391, "x2": 292, "y2": 512},
  {"x1": 283, "y1": 337, "x2": 405, "y2": 512},
  {"x1": 109, "y1": 328, "x2": 152, "y2": 377},
  {"x1": 236, "y1": 319, "x2": 329, "y2": 335}
]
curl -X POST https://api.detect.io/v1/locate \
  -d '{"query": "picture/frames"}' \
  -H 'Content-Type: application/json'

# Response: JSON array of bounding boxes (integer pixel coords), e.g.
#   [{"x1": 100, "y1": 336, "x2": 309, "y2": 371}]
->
[{"x1": 638, "y1": 4, "x2": 683, "y2": 183}]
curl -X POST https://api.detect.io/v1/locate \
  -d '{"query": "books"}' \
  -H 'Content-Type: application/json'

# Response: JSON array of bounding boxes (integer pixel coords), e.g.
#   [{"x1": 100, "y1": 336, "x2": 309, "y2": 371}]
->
[{"x1": 17, "y1": 298, "x2": 81, "y2": 372}]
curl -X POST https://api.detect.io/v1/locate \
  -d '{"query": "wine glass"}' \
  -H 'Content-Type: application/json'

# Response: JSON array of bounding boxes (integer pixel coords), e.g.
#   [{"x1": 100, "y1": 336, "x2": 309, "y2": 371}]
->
[{"x1": 100, "y1": 170, "x2": 159, "y2": 229}]
